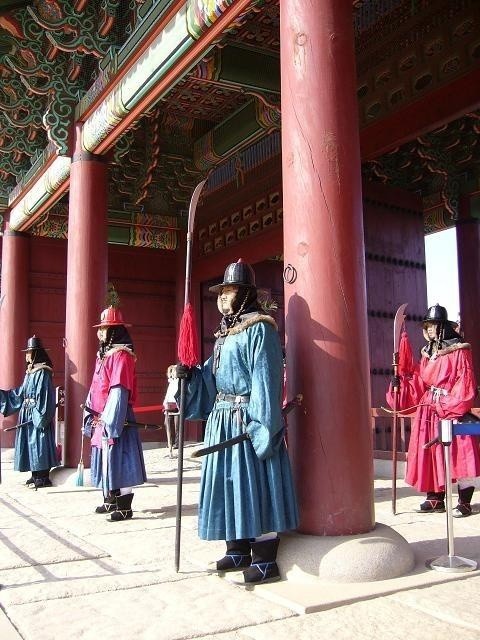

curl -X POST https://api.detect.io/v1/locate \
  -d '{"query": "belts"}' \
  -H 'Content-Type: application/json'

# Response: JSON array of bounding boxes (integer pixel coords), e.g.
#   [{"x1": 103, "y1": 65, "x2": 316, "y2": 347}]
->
[
  {"x1": 22, "y1": 398, "x2": 36, "y2": 403},
  {"x1": 213, "y1": 392, "x2": 251, "y2": 404}
]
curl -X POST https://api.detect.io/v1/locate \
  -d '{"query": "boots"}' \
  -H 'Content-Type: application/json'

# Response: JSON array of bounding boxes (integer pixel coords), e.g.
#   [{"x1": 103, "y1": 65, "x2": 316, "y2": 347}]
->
[
  {"x1": 231, "y1": 536, "x2": 283, "y2": 587},
  {"x1": 206, "y1": 536, "x2": 255, "y2": 572},
  {"x1": 26, "y1": 468, "x2": 53, "y2": 489},
  {"x1": 414, "y1": 490, "x2": 446, "y2": 515},
  {"x1": 452, "y1": 483, "x2": 476, "y2": 519},
  {"x1": 106, "y1": 493, "x2": 135, "y2": 522},
  {"x1": 96, "y1": 488, "x2": 121, "y2": 514}
]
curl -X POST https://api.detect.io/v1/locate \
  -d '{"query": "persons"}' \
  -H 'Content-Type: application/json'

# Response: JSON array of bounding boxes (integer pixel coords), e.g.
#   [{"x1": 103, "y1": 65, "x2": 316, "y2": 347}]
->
[
  {"x1": 162, "y1": 363, "x2": 181, "y2": 460},
  {"x1": 175, "y1": 262, "x2": 301, "y2": 588},
  {"x1": 84, "y1": 305, "x2": 148, "y2": 522},
  {"x1": 0, "y1": 334, "x2": 63, "y2": 489},
  {"x1": 384, "y1": 305, "x2": 480, "y2": 518}
]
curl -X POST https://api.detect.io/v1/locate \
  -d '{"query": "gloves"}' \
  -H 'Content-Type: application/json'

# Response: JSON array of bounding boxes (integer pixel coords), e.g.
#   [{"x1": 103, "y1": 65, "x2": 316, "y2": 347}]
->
[
  {"x1": 390, "y1": 375, "x2": 400, "y2": 388},
  {"x1": 175, "y1": 362, "x2": 192, "y2": 382}
]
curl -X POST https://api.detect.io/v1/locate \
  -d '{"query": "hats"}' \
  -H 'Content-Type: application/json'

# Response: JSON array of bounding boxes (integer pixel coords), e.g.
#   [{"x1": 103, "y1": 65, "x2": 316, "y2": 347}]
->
[
  {"x1": 416, "y1": 304, "x2": 460, "y2": 329},
  {"x1": 20, "y1": 334, "x2": 51, "y2": 352},
  {"x1": 91, "y1": 304, "x2": 132, "y2": 329},
  {"x1": 207, "y1": 257, "x2": 270, "y2": 293}
]
